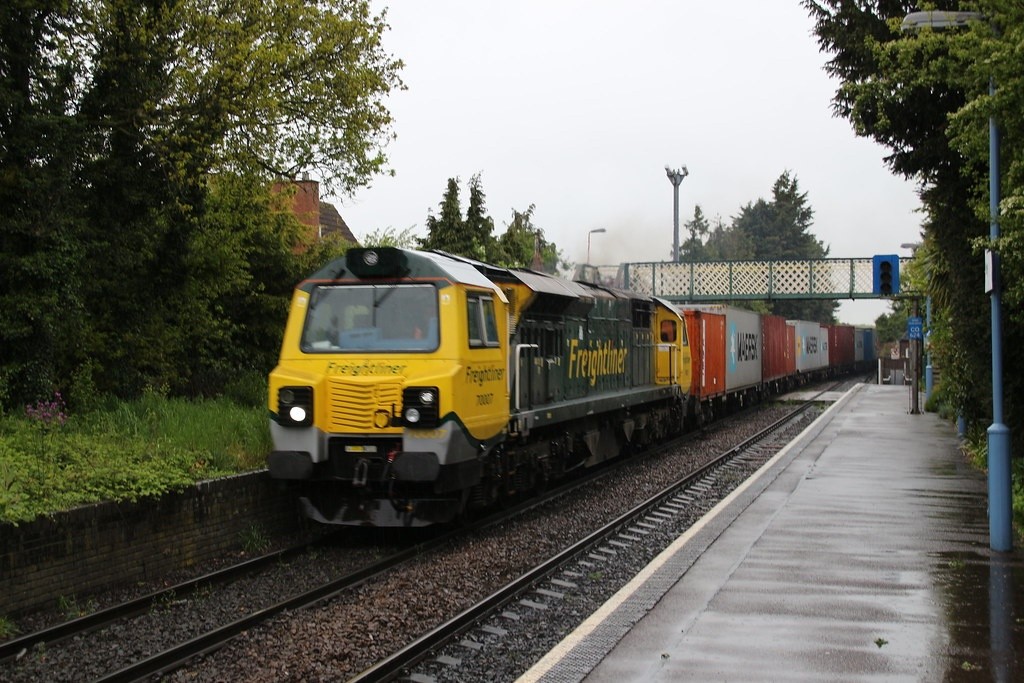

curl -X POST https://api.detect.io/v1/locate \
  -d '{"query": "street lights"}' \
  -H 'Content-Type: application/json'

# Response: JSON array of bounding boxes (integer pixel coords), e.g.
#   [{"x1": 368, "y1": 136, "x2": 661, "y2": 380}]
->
[
  {"x1": 900, "y1": 243, "x2": 934, "y2": 398},
  {"x1": 902, "y1": 10, "x2": 1013, "y2": 555},
  {"x1": 587, "y1": 228, "x2": 606, "y2": 265}
]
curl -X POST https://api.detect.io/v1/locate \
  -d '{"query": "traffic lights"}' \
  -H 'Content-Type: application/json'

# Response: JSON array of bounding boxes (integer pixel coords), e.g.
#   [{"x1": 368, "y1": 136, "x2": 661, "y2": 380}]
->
[{"x1": 873, "y1": 254, "x2": 899, "y2": 295}]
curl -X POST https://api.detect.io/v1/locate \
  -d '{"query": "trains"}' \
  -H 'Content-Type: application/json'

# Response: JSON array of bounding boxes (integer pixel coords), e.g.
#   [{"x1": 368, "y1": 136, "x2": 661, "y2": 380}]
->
[{"x1": 268, "y1": 243, "x2": 879, "y2": 530}]
